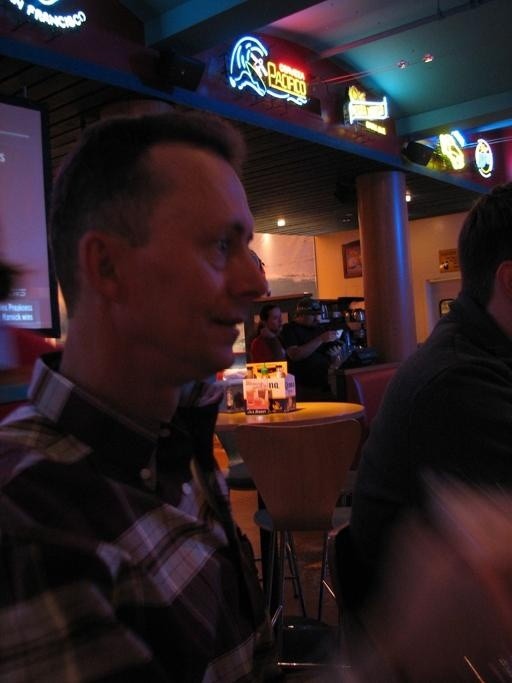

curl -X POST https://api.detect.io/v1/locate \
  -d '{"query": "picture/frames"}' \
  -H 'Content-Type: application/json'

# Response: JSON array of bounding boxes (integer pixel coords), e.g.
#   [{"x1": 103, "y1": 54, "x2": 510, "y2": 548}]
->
[{"x1": 341, "y1": 238, "x2": 362, "y2": 279}]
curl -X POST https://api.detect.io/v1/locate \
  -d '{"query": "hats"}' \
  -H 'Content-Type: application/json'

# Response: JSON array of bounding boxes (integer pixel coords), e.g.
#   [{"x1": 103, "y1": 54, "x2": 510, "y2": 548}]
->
[{"x1": 297, "y1": 299, "x2": 324, "y2": 315}]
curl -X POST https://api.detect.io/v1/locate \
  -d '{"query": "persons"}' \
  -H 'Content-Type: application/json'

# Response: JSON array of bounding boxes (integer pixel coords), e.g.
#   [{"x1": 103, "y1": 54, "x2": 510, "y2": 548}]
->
[
  {"x1": 278, "y1": 297, "x2": 345, "y2": 402},
  {"x1": 248, "y1": 300, "x2": 287, "y2": 361},
  {"x1": 0, "y1": 106, "x2": 512, "y2": 682},
  {"x1": 0, "y1": 256, "x2": 58, "y2": 419},
  {"x1": 329, "y1": 178, "x2": 511, "y2": 612}
]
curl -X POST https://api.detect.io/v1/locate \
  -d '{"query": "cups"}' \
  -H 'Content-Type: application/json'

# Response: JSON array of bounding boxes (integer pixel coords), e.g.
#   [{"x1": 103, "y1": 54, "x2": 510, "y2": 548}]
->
[{"x1": 246, "y1": 388, "x2": 266, "y2": 406}]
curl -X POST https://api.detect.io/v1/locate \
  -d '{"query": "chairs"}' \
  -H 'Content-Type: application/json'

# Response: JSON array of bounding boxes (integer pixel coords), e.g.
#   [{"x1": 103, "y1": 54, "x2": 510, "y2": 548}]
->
[{"x1": 234, "y1": 417, "x2": 362, "y2": 613}]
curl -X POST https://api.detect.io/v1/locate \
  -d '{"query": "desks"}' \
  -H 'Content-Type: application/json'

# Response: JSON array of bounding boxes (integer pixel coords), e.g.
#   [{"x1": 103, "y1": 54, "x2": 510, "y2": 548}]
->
[{"x1": 212, "y1": 400, "x2": 367, "y2": 673}]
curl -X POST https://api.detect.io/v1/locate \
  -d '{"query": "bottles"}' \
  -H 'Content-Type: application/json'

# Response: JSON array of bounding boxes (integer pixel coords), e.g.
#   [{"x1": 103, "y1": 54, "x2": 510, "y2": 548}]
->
[
  {"x1": 276, "y1": 364, "x2": 284, "y2": 378},
  {"x1": 261, "y1": 362, "x2": 270, "y2": 379},
  {"x1": 247, "y1": 366, "x2": 257, "y2": 378}
]
